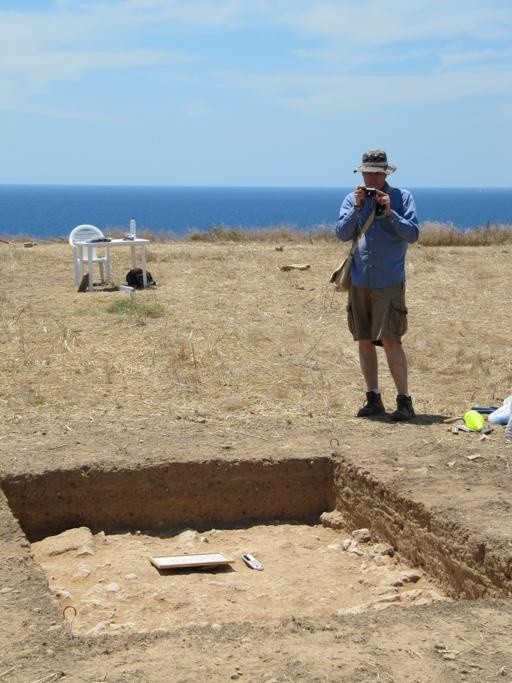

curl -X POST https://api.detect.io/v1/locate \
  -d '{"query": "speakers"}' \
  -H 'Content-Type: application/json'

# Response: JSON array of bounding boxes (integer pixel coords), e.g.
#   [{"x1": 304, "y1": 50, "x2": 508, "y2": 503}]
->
[
  {"x1": 390, "y1": 395, "x2": 417, "y2": 421},
  {"x1": 357, "y1": 391, "x2": 386, "y2": 417}
]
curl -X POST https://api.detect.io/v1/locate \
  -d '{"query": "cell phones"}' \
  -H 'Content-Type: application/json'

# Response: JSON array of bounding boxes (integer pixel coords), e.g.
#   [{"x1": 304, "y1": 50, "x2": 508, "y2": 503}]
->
[{"x1": 362, "y1": 188, "x2": 376, "y2": 198}]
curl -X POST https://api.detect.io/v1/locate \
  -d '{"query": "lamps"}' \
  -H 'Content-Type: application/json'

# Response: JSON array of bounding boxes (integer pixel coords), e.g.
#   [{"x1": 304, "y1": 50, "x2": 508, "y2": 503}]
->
[{"x1": 68, "y1": 224, "x2": 110, "y2": 291}]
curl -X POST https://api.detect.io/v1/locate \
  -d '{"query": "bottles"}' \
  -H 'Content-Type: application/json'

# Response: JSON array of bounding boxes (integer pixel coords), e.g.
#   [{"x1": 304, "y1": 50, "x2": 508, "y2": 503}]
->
[{"x1": 353, "y1": 149, "x2": 397, "y2": 175}]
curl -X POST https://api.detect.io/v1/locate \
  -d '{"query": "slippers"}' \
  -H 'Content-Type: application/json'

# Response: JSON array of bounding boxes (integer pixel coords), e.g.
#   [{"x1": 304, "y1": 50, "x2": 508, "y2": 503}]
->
[{"x1": 125, "y1": 268, "x2": 155, "y2": 289}]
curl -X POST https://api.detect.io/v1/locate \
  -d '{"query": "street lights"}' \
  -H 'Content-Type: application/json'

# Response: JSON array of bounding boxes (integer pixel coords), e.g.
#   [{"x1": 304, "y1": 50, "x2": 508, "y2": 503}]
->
[{"x1": 331, "y1": 253, "x2": 354, "y2": 292}]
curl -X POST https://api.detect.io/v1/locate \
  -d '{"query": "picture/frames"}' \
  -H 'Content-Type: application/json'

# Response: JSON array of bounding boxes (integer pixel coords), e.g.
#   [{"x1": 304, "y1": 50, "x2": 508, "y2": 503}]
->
[{"x1": 73, "y1": 238, "x2": 150, "y2": 293}]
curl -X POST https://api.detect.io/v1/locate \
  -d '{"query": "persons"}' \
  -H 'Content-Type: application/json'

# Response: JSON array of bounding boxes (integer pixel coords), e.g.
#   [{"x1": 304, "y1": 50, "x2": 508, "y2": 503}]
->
[{"x1": 334, "y1": 147, "x2": 421, "y2": 420}]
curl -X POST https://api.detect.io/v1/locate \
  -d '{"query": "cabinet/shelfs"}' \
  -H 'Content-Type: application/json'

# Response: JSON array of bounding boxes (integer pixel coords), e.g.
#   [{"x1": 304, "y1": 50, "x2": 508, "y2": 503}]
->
[
  {"x1": 129, "y1": 217, "x2": 137, "y2": 239},
  {"x1": 119, "y1": 285, "x2": 135, "y2": 293}
]
